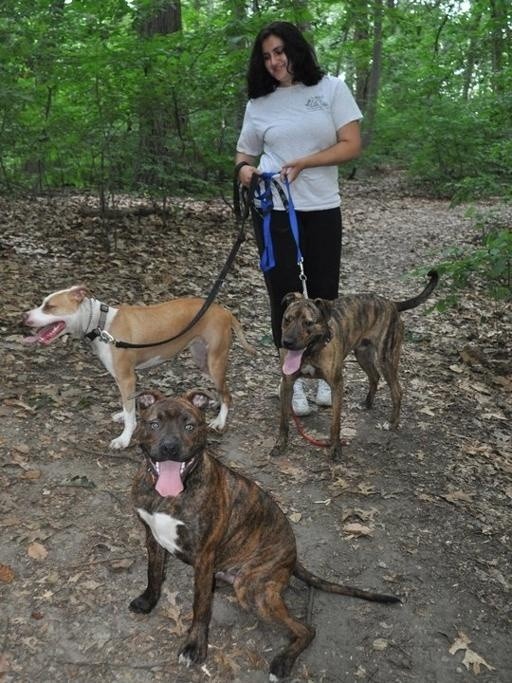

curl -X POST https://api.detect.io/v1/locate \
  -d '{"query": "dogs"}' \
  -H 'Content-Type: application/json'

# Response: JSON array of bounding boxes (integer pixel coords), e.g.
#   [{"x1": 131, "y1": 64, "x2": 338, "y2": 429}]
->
[
  {"x1": 270, "y1": 269, "x2": 439, "y2": 461},
  {"x1": 126, "y1": 388, "x2": 405, "y2": 683},
  {"x1": 21, "y1": 283, "x2": 257, "y2": 450}
]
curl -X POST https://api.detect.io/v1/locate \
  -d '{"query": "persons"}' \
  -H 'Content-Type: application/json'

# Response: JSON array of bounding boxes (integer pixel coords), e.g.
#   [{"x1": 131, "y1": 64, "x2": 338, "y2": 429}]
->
[{"x1": 236, "y1": 21, "x2": 365, "y2": 417}]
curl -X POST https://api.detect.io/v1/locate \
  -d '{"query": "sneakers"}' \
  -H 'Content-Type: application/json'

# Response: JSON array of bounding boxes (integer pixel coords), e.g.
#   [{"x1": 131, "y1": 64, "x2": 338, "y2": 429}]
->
[
  {"x1": 278, "y1": 376, "x2": 311, "y2": 416},
  {"x1": 316, "y1": 378, "x2": 332, "y2": 406}
]
